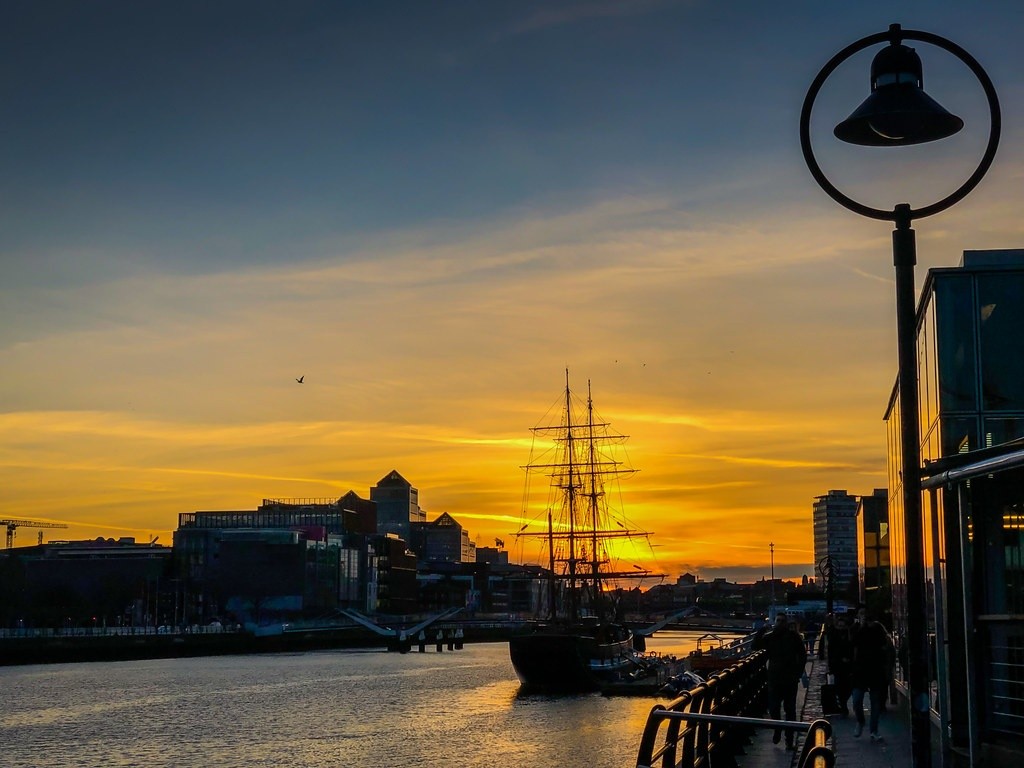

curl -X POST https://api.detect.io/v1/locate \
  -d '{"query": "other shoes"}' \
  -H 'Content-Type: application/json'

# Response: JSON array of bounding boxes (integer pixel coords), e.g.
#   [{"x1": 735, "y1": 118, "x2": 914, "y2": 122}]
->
[
  {"x1": 772, "y1": 726, "x2": 782, "y2": 743},
  {"x1": 853, "y1": 721, "x2": 865, "y2": 738},
  {"x1": 785, "y1": 737, "x2": 793, "y2": 749},
  {"x1": 870, "y1": 733, "x2": 883, "y2": 744}
]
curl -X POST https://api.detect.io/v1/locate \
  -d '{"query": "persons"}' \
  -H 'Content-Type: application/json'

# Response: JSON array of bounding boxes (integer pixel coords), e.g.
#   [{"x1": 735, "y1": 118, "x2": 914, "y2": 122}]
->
[
  {"x1": 754, "y1": 606, "x2": 810, "y2": 752},
  {"x1": 818, "y1": 603, "x2": 894, "y2": 745}
]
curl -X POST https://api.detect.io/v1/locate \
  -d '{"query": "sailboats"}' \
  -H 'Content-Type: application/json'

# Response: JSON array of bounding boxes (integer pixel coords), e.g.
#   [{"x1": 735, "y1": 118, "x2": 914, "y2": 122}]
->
[{"x1": 505, "y1": 365, "x2": 671, "y2": 692}]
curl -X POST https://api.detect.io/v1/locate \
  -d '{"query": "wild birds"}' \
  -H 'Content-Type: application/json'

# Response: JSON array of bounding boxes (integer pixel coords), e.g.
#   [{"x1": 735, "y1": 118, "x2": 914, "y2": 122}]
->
[{"x1": 295, "y1": 376, "x2": 304, "y2": 383}]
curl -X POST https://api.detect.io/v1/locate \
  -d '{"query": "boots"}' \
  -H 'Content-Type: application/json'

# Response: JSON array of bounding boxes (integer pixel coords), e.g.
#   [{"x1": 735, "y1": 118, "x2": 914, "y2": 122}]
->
[{"x1": 879, "y1": 697, "x2": 887, "y2": 713}]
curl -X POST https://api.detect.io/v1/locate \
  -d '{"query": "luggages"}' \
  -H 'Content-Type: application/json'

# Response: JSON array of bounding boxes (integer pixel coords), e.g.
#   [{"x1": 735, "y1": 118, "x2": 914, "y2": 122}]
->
[{"x1": 822, "y1": 672, "x2": 843, "y2": 716}]
